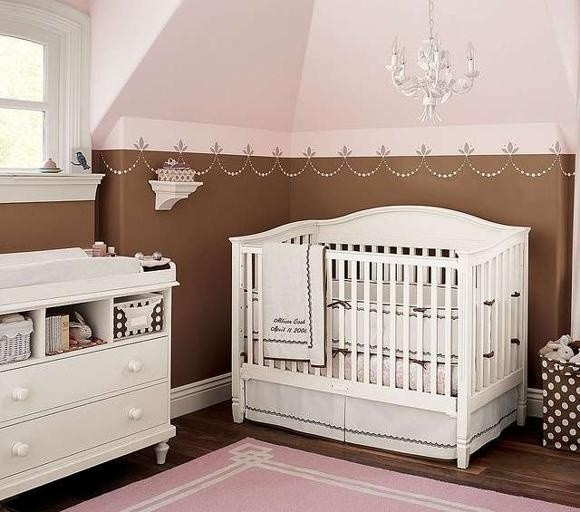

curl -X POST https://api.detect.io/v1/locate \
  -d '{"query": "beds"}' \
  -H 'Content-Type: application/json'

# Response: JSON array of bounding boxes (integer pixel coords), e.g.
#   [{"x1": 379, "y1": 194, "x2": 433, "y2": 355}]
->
[{"x1": 223, "y1": 202, "x2": 529, "y2": 468}]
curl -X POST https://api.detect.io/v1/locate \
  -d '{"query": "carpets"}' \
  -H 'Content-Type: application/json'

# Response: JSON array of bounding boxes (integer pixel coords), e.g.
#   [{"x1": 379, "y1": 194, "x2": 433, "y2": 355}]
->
[{"x1": 51, "y1": 434, "x2": 574, "y2": 511}]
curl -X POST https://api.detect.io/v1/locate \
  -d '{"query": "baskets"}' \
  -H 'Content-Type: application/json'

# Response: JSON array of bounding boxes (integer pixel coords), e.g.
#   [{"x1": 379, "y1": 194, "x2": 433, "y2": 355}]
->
[{"x1": 0, "y1": 317, "x2": 33, "y2": 365}]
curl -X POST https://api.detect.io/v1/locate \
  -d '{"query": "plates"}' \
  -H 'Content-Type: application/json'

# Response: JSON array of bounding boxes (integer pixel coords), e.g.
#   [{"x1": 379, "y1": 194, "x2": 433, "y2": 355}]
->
[{"x1": 36, "y1": 168, "x2": 64, "y2": 173}]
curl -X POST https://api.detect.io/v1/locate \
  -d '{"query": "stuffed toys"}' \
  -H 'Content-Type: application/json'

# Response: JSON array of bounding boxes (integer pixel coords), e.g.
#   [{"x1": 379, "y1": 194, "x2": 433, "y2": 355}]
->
[
  {"x1": 69, "y1": 311, "x2": 92, "y2": 345},
  {"x1": 543, "y1": 340, "x2": 580, "y2": 364}
]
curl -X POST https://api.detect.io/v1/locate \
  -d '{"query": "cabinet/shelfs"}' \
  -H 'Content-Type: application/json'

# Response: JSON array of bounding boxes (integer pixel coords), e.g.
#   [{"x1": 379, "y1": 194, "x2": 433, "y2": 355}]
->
[{"x1": 0, "y1": 278, "x2": 180, "y2": 502}]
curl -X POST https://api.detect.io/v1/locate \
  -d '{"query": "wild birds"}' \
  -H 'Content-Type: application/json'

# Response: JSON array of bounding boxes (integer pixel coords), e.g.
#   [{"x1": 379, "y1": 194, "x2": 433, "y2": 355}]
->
[{"x1": 75, "y1": 152, "x2": 91, "y2": 170}]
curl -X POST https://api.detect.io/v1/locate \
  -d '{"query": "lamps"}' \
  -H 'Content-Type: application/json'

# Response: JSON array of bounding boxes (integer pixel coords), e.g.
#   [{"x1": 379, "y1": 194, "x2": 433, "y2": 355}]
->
[{"x1": 387, "y1": 0, "x2": 477, "y2": 124}]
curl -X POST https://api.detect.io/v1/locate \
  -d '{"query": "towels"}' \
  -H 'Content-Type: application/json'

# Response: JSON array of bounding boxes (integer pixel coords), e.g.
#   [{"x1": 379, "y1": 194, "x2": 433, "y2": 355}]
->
[{"x1": 256, "y1": 240, "x2": 330, "y2": 367}]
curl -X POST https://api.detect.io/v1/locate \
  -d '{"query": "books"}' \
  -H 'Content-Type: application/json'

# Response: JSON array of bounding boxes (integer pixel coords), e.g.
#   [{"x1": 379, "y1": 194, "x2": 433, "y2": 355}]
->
[{"x1": 45, "y1": 312, "x2": 70, "y2": 354}]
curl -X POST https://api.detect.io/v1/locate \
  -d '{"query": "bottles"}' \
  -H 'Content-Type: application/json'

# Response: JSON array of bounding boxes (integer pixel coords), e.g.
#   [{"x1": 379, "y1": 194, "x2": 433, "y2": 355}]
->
[{"x1": 92, "y1": 241, "x2": 106, "y2": 257}]
